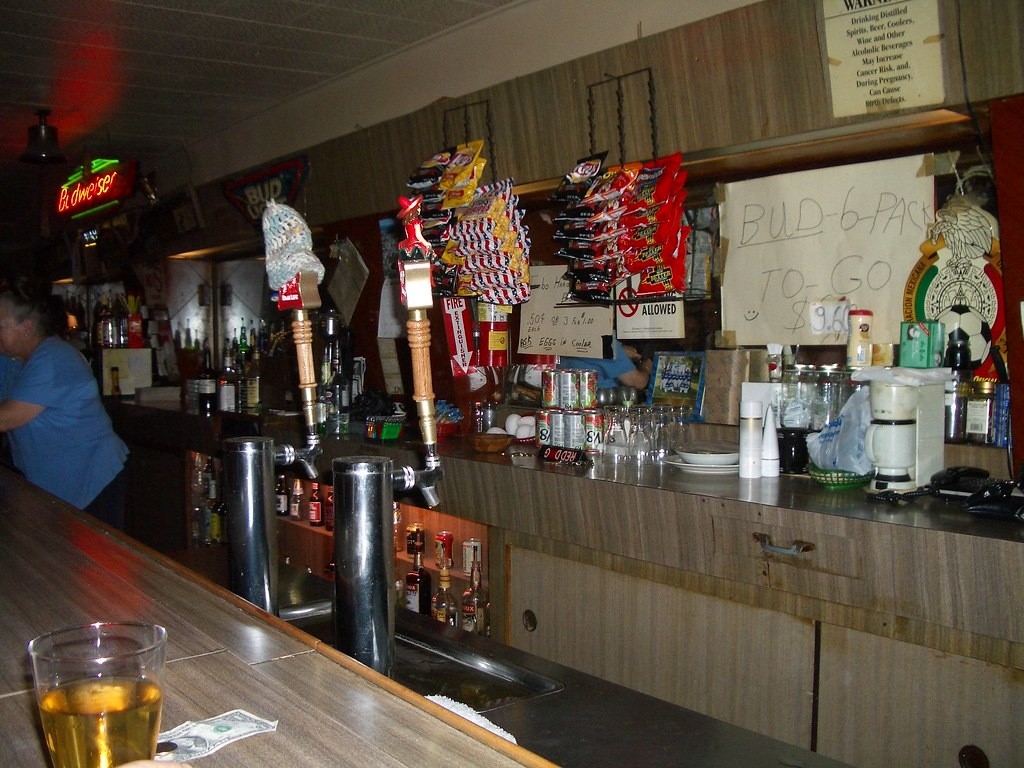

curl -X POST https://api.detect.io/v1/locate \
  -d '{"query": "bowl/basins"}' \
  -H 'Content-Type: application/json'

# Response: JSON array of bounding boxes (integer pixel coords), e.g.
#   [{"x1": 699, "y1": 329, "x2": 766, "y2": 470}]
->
[{"x1": 674, "y1": 449, "x2": 739, "y2": 465}]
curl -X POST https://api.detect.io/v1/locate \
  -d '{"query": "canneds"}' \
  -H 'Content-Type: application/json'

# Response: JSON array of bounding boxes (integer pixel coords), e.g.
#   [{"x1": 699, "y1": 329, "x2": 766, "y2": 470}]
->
[
  {"x1": 535, "y1": 368, "x2": 614, "y2": 454},
  {"x1": 463, "y1": 538, "x2": 482, "y2": 576},
  {"x1": 966, "y1": 381, "x2": 995, "y2": 444},
  {"x1": 406, "y1": 522, "x2": 425, "y2": 559},
  {"x1": 434, "y1": 531, "x2": 453, "y2": 569}
]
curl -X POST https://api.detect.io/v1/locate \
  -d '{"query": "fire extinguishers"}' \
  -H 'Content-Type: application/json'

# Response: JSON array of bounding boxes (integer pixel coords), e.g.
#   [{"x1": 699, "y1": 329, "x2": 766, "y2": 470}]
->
[{"x1": 470, "y1": 297, "x2": 510, "y2": 366}]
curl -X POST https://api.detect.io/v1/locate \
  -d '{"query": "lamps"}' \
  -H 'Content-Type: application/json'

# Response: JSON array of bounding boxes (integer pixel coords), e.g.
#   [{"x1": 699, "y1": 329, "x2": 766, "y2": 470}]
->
[{"x1": 16, "y1": 110, "x2": 67, "y2": 165}]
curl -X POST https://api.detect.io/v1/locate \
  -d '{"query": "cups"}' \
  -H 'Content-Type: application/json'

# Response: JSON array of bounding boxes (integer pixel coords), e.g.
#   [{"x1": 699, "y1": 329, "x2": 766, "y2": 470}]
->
[{"x1": 28, "y1": 620, "x2": 169, "y2": 768}]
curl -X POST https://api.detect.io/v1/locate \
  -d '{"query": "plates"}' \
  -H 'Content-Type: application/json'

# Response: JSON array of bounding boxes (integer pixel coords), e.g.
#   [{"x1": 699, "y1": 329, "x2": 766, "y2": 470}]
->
[{"x1": 663, "y1": 454, "x2": 739, "y2": 475}]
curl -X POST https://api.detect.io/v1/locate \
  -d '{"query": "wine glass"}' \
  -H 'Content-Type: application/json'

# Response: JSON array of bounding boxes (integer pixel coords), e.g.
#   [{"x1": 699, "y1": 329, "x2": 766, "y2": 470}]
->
[{"x1": 603, "y1": 405, "x2": 689, "y2": 467}]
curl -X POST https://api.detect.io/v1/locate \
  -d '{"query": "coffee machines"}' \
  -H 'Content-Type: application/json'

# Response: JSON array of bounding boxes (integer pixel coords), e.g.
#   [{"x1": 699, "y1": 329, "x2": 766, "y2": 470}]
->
[{"x1": 863, "y1": 368, "x2": 946, "y2": 505}]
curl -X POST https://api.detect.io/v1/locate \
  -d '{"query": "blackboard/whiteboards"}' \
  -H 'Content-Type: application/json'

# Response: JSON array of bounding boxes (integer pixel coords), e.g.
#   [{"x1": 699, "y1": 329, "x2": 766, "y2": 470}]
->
[
  {"x1": 515, "y1": 263, "x2": 605, "y2": 357},
  {"x1": 715, "y1": 151, "x2": 936, "y2": 348}
]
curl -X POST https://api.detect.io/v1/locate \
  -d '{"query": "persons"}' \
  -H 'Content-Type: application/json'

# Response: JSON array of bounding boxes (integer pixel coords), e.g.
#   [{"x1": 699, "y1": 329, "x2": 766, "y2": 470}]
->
[
  {"x1": 560, "y1": 313, "x2": 702, "y2": 389},
  {"x1": 0, "y1": 278, "x2": 130, "y2": 532}
]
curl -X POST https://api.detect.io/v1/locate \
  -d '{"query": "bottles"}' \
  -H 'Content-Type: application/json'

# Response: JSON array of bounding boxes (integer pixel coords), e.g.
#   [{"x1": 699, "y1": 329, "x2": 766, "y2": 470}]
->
[
  {"x1": 308, "y1": 483, "x2": 324, "y2": 527},
  {"x1": 393, "y1": 502, "x2": 404, "y2": 552},
  {"x1": 430, "y1": 569, "x2": 458, "y2": 628},
  {"x1": 781, "y1": 364, "x2": 862, "y2": 475},
  {"x1": 173, "y1": 326, "x2": 262, "y2": 414},
  {"x1": 461, "y1": 560, "x2": 489, "y2": 637},
  {"x1": 332, "y1": 338, "x2": 351, "y2": 435},
  {"x1": 326, "y1": 486, "x2": 334, "y2": 531},
  {"x1": 315, "y1": 343, "x2": 335, "y2": 440},
  {"x1": 739, "y1": 400, "x2": 763, "y2": 479},
  {"x1": 846, "y1": 310, "x2": 874, "y2": 372},
  {"x1": 289, "y1": 477, "x2": 306, "y2": 521},
  {"x1": 275, "y1": 473, "x2": 290, "y2": 517},
  {"x1": 63, "y1": 289, "x2": 129, "y2": 348},
  {"x1": 964, "y1": 381, "x2": 996, "y2": 445},
  {"x1": 190, "y1": 453, "x2": 226, "y2": 549},
  {"x1": 471, "y1": 401, "x2": 497, "y2": 434},
  {"x1": 404, "y1": 542, "x2": 432, "y2": 615}
]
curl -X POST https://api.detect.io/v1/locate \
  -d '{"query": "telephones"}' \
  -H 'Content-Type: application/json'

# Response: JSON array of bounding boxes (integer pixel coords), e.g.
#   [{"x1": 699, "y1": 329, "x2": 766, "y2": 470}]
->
[{"x1": 931, "y1": 465, "x2": 1017, "y2": 499}]
